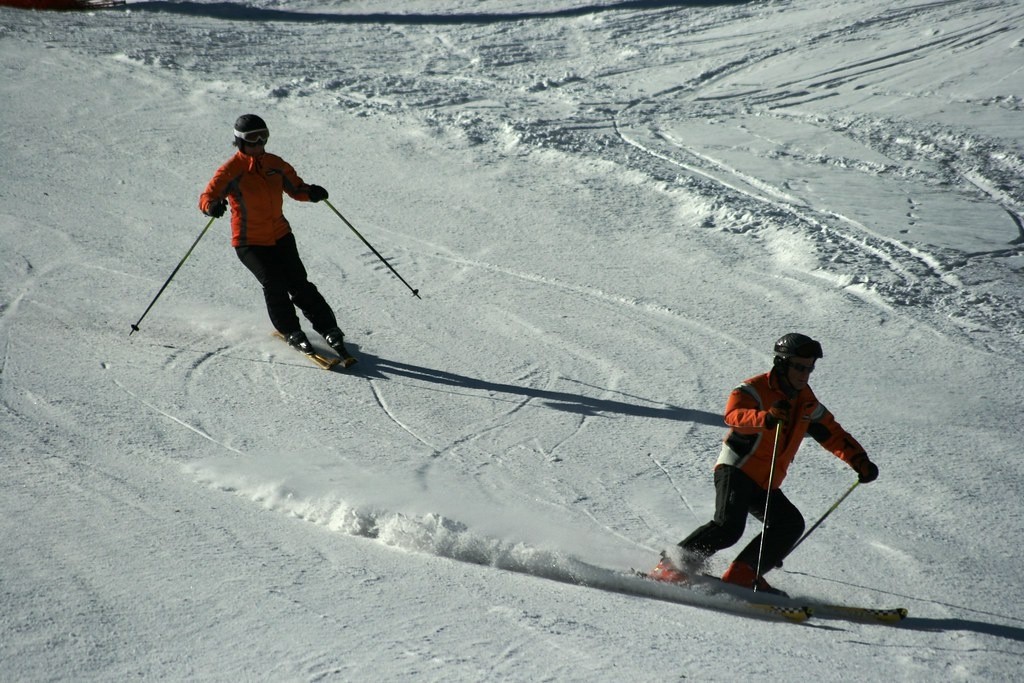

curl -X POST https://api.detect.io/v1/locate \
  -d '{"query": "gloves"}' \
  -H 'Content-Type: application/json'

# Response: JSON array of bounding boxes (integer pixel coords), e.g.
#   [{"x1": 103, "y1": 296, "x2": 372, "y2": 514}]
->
[
  {"x1": 309, "y1": 184, "x2": 329, "y2": 202},
  {"x1": 209, "y1": 199, "x2": 228, "y2": 218},
  {"x1": 858, "y1": 460, "x2": 878, "y2": 483},
  {"x1": 764, "y1": 400, "x2": 791, "y2": 429}
]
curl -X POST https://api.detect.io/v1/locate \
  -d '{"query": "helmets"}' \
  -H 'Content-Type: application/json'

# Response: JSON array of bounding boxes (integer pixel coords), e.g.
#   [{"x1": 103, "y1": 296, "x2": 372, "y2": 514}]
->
[
  {"x1": 234, "y1": 114, "x2": 270, "y2": 141},
  {"x1": 773, "y1": 333, "x2": 823, "y2": 363}
]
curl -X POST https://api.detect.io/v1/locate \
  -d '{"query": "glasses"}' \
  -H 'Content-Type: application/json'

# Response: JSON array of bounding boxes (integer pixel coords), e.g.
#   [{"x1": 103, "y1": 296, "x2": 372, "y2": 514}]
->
[
  {"x1": 794, "y1": 363, "x2": 814, "y2": 372},
  {"x1": 243, "y1": 129, "x2": 267, "y2": 143}
]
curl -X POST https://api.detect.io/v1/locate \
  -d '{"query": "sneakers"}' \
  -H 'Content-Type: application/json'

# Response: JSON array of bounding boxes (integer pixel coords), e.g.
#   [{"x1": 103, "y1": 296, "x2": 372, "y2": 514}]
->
[
  {"x1": 323, "y1": 327, "x2": 344, "y2": 348},
  {"x1": 721, "y1": 561, "x2": 789, "y2": 598},
  {"x1": 286, "y1": 331, "x2": 313, "y2": 355},
  {"x1": 650, "y1": 560, "x2": 689, "y2": 583}
]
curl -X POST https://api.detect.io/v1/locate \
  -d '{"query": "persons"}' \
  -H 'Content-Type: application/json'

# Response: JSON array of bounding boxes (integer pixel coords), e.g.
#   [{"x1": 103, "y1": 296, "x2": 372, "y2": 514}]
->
[
  {"x1": 199, "y1": 113, "x2": 346, "y2": 355},
  {"x1": 650, "y1": 333, "x2": 879, "y2": 591}
]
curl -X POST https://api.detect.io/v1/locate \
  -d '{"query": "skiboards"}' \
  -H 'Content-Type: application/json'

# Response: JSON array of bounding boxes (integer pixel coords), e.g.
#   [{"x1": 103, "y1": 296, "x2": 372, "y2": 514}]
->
[
  {"x1": 567, "y1": 555, "x2": 909, "y2": 627},
  {"x1": 270, "y1": 328, "x2": 360, "y2": 371}
]
